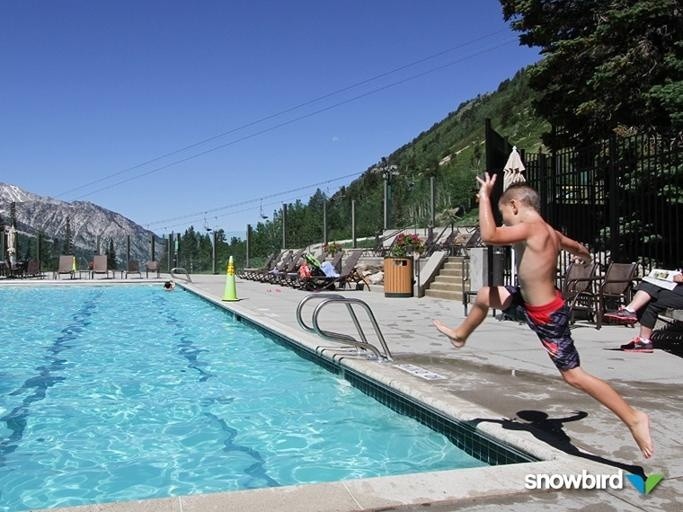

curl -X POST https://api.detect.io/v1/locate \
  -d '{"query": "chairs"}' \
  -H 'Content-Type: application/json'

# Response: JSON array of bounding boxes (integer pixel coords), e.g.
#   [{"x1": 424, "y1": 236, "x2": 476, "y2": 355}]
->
[
  {"x1": 498, "y1": 259, "x2": 636, "y2": 331},
  {"x1": 0, "y1": 253, "x2": 161, "y2": 279},
  {"x1": 234, "y1": 247, "x2": 372, "y2": 293}
]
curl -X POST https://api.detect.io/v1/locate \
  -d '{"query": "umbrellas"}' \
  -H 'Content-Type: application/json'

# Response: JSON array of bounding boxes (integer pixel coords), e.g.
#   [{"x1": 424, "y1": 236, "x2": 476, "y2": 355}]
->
[
  {"x1": 503, "y1": 145, "x2": 527, "y2": 288},
  {"x1": 7, "y1": 229, "x2": 16, "y2": 268}
]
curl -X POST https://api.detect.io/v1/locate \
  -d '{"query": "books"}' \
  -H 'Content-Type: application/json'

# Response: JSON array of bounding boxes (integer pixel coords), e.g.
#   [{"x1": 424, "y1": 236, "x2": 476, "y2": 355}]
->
[{"x1": 641, "y1": 269, "x2": 682, "y2": 291}]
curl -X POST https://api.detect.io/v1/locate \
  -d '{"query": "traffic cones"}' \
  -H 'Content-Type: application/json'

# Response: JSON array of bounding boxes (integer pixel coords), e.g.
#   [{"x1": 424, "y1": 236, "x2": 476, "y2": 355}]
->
[{"x1": 219, "y1": 253, "x2": 241, "y2": 301}]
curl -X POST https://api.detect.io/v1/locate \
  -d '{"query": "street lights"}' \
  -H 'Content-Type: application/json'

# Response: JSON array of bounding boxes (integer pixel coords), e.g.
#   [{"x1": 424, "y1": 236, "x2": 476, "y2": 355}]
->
[{"x1": 369, "y1": 162, "x2": 400, "y2": 231}]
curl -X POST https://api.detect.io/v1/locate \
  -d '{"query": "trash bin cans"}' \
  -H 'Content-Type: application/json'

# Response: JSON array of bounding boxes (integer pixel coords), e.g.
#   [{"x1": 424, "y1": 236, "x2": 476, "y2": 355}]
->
[{"x1": 384, "y1": 256, "x2": 413, "y2": 296}]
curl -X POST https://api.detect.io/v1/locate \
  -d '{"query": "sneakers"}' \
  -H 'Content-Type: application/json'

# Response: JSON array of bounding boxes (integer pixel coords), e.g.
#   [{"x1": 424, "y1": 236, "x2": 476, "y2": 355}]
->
[
  {"x1": 604, "y1": 304, "x2": 637, "y2": 323},
  {"x1": 621, "y1": 336, "x2": 653, "y2": 353}
]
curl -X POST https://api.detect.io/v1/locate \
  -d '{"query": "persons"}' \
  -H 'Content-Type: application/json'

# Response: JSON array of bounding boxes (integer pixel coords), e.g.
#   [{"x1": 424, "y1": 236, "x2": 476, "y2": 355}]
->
[
  {"x1": 603, "y1": 274, "x2": 683, "y2": 353},
  {"x1": 434, "y1": 171, "x2": 653, "y2": 459},
  {"x1": 165, "y1": 282, "x2": 172, "y2": 289}
]
